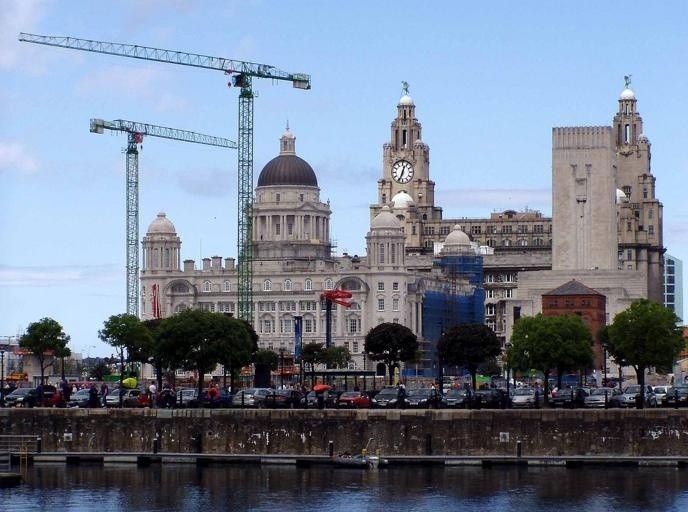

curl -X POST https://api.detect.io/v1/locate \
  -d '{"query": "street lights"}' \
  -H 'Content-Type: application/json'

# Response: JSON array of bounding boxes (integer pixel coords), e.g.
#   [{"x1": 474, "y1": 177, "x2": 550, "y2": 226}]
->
[
  {"x1": 0, "y1": 349, "x2": 6, "y2": 408},
  {"x1": 504, "y1": 342, "x2": 511, "y2": 409},
  {"x1": 617, "y1": 359, "x2": 627, "y2": 390},
  {"x1": 602, "y1": 343, "x2": 610, "y2": 409},
  {"x1": 279, "y1": 346, "x2": 285, "y2": 389}
]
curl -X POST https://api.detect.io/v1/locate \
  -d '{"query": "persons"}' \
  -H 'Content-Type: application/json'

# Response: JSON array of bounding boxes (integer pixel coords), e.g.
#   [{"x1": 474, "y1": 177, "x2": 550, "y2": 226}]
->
[
  {"x1": 148, "y1": 380, "x2": 220, "y2": 407},
  {"x1": 34, "y1": 378, "x2": 109, "y2": 408},
  {"x1": 397, "y1": 378, "x2": 558, "y2": 408}
]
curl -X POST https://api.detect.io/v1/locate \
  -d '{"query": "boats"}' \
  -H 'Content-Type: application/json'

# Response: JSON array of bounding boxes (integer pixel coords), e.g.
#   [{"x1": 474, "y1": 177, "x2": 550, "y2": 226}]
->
[{"x1": 332, "y1": 437, "x2": 389, "y2": 469}]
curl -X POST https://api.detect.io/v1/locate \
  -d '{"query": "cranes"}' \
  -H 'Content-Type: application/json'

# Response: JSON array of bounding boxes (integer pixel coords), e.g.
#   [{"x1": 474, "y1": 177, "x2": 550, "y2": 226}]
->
[
  {"x1": 18, "y1": 29, "x2": 312, "y2": 389},
  {"x1": 89, "y1": 119, "x2": 238, "y2": 379}
]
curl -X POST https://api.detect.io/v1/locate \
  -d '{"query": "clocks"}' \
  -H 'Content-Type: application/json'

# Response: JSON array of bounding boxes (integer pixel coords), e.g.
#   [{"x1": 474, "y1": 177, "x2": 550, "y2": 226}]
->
[{"x1": 390, "y1": 159, "x2": 414, "y2": 185}]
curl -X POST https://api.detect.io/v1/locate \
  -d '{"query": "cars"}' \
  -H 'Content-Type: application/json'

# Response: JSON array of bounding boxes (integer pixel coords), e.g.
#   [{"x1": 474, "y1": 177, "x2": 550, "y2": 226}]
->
[
  {"x1": 660, "y1": 387, "x2": 687, "y2": 408},
  {"x1": 403, "y1": 386, "x2": 669, "y2": 408},
  {"x1": 69, "y1": 386, "x2": 337, "y2": 408},
  {"x1": 3, "y1": 388, "x2": 38, "y2": 409},
  {"x1": 338, "y1": 391, "x2": 369, "y2": 408}
]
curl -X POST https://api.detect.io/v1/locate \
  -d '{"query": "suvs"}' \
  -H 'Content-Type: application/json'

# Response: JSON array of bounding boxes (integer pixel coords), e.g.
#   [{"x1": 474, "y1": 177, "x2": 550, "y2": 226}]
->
[{"x1": 371, "y1": 386, "x2": 405, "y2": 408}]
